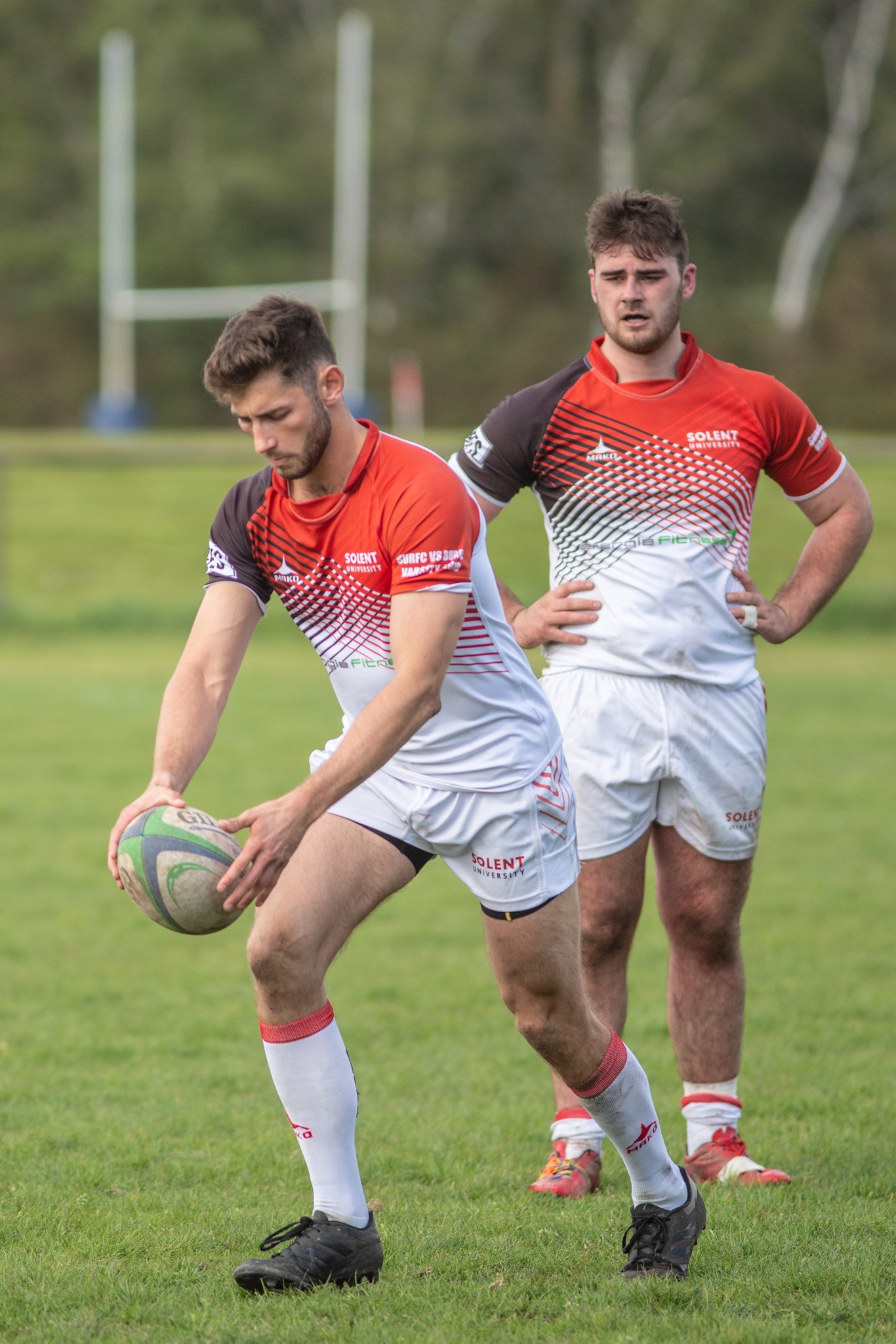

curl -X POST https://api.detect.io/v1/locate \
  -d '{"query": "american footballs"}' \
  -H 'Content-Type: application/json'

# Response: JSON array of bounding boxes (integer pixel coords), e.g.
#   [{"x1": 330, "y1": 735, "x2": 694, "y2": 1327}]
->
[{"x1": 117, "y1": 805, "x2": 250, "y2": 935}]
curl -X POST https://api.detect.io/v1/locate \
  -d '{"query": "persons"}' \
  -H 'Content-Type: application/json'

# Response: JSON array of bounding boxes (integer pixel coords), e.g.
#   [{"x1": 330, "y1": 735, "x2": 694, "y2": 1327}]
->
[
  {"x1": 443, "y1": 189, "x2": 878, "y2": 1199},
  {"x1": 105, "y1": 288, "x2": 704, "y2": 1291}
]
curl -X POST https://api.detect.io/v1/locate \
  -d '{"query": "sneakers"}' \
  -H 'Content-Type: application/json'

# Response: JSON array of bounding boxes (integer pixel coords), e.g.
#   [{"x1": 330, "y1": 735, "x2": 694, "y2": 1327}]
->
[
  {"x1": 619, "y1": 1165, "x2": 707, "y2": 1279},
  {"x1": 526, "y1": 1137, "x2": 602, "y2": 1197},
  {"x1": 233, "y1": 1210, "x2": 384, "y2": 1294},
  {"x1": 687, "y1": 1125, "x2": 792, "y2": 1187}
]
